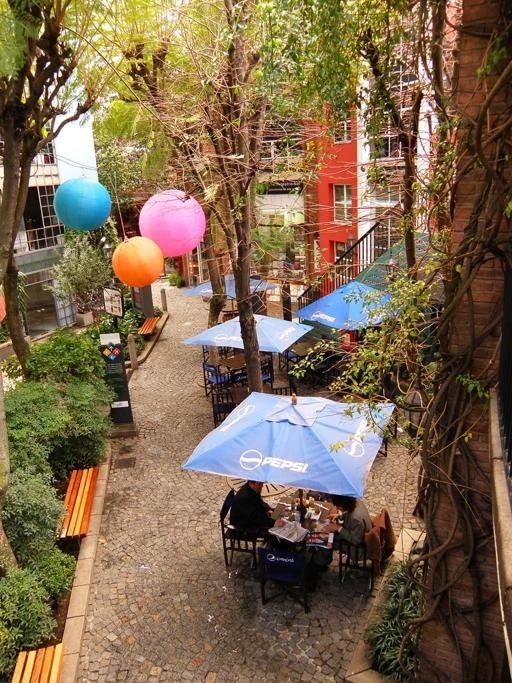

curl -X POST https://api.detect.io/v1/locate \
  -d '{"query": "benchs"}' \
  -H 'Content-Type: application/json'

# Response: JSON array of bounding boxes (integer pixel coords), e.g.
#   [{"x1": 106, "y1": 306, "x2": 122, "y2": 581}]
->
[
  {"x1": 57, "y1": 465, "x2": 99, "y2": 552},
  {"x1": 137, "y1": 315, "x2": 161, "y2": 342},
  {"x1": 10, "y1": 642, "x2": 66, "y2": 683}
]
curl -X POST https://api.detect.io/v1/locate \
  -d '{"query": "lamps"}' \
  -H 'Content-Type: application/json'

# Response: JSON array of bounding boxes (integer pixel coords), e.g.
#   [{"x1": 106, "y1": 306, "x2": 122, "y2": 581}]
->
[{"x1": 400, "y1": 366, "x2": 431, "y2": 440}]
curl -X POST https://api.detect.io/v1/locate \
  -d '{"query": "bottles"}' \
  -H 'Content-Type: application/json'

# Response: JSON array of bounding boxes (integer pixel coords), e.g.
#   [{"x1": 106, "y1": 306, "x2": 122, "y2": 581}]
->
[{"x1": 289, "y1": 488, "x2": 307, "y2": 525}]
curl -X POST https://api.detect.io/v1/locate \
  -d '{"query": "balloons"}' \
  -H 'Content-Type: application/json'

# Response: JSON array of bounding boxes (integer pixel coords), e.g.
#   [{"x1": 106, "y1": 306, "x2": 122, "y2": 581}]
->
[
  {"x1": 138, "y1": 189, "x2": 207, "y2": 258},
  {"x1": 52, "y1": 176, "x2": 113, "y2": 231},
  {"x1": 111, "y1": 236, "x2": 165, "y2": 288}
]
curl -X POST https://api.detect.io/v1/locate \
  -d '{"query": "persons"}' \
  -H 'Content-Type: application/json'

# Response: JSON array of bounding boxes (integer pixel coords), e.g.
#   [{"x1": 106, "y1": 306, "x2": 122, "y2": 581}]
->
[
  {"x1": 321, "y1": 495, "x2": 372, "y2": 579},
  {"x1": 230, "y1": 480, "x2": 276, "y2": 533}
]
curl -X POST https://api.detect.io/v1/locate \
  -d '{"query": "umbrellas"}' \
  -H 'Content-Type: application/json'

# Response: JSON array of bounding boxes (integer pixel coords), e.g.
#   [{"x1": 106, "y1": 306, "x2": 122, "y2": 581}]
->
[
  {"x1": 295, "y1": 281, "x2": 405, "y2": 336},
  {"x1": 182, "y1": 389, "x2": 397, "y2": 530},
  {"x1": 184, "y1": 273, "x2": 278, "y2": 317},
  {"x1": 182, "y1": 314, "x2": 313, "y2": 354}
]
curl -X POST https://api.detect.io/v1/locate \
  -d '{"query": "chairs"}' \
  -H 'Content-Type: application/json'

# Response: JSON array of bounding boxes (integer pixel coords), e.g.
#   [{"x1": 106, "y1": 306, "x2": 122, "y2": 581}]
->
[
  {"x1": 203, "y1": 337, "x2": 396, "y2": 459},
  {"x1": 216, "y1": 488, "x2": 394, "y2": 613}
]
